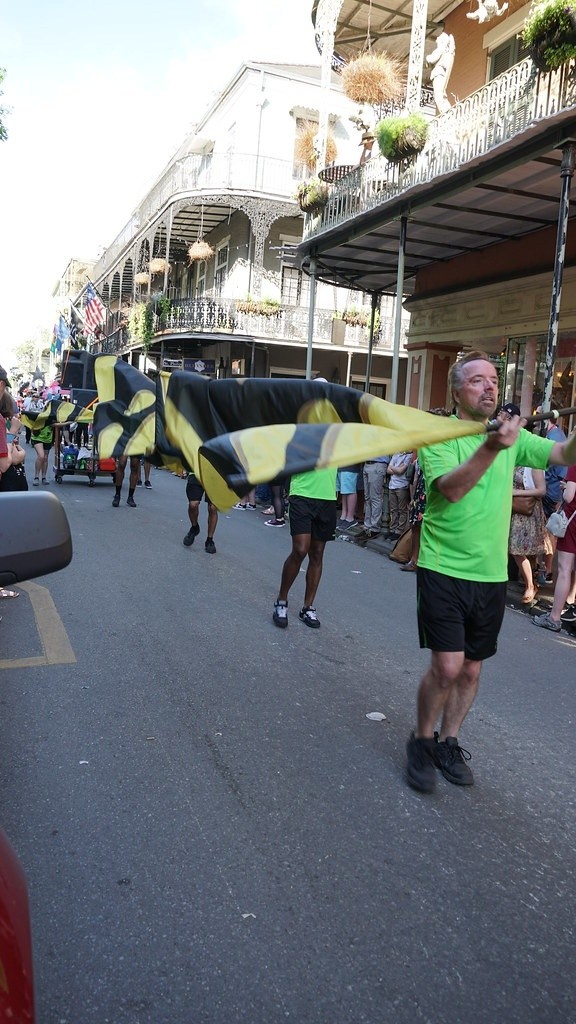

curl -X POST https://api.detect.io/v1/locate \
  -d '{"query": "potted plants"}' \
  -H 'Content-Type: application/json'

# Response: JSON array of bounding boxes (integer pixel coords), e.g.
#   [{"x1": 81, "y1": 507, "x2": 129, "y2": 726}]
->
[
  {"x1": 518, "y1": 1, "x2": 576, "y2": 74},
  {"x1": 127, "y1": 296, "x2": 169, "y2": 352},
  {"x1": 296, "y1": 181, "x2": 329, "y2": 213},
  {"x1": 375, "y1": 111, "x2": 426, "y2": 161}
]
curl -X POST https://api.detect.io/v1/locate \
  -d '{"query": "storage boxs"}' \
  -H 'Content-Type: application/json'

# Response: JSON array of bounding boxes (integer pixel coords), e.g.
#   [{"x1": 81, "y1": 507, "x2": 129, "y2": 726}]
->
[{"x1": 99, "y1": 457, "x2": 116, "y2": 470}]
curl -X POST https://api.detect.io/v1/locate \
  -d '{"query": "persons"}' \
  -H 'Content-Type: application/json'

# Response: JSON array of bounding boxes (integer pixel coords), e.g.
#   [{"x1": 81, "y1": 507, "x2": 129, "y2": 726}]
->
[
  {"x1": 272, "y1": 376, "x2": 338, "y2": 628},
  {"x1": 136, "y1": 461, "x2": 152, "y2": 489},
  {"x1": 112, "y1": 453, "x2": 141, "y2": 507},
  {"x1": 32, "y1": 421, "x2": 73, "y2": 486},
  {"x1": 183, "y1": 471, "x2": 218, "y2": 553},
  {"x1": 426, "y1": 32, "x2": 454, "y2": 113},
  {"x1": 0, "y1": 363, "x2": 71, "y2": 598},
  {"x1": 234, "y1": 399, "x2": 576, "y2": 632},
  {"x1": 404, "y1": 351, "x2": 576, "y2": 794}
]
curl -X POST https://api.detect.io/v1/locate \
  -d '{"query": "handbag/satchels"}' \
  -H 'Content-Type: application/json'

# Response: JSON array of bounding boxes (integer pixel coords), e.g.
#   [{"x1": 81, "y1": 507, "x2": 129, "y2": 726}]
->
[
  {"x1": 389, "y1": 526, "x2": 414, "y2": 563},
  {"x1": 512, "y1": 496, "x2": 535, "y2": 515},
  {"x1": 545, "y1": 510, "x2": 569, "y2": 538},
  {"x1": 0, "y1": 459, "x2": 28, "y2": 492}
]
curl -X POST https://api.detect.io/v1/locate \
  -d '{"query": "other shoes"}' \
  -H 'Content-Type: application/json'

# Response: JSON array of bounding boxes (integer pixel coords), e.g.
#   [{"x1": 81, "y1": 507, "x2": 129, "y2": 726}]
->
[
  {"x1": 136, "y1": 479, "x2": 142, "y2": 487},
  {"x1": 112, "y1": 495, "x2": 120, "y2": 507},
  {"x1": 400, "y1": 562, "x2": 417, "y2": 571},
  {"x1": 232, "y1": 500, "x2": 403, "y2": 544},
  {"x1": 144, "y1": 479, "x2": 152, "y2": 488},
  {"x1": 0, "y1": 588, "x2": 18, "y2": 598},
  {"x1": 126, "y1": 497, "x2": 136, "y2": 507},
  {"x1": 24, "y1": 436, "x2": 94, "y2": 485}
]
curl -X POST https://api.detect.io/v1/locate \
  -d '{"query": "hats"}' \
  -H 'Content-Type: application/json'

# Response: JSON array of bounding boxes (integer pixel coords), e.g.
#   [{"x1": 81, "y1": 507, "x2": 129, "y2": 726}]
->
[
  {"x1": 501, "y1": 402, "x2": 520, "y2": 417},
  {"x1": 0, "y1": 365, "x2": 12, "y2": 388}
]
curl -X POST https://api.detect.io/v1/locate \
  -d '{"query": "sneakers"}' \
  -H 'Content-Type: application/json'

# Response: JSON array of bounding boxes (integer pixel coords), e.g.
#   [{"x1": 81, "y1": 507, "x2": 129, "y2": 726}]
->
[
  {"x1": 205, "y1": 537, "x2": 216, "y2": 553},
  {"x1": 273, "y1": 599, "x2": 289, "y2": 627},
  {"x1": 183, "y1": 523, "x2": 200, "y2": 546},
  {"x1": 406, "y1": 731, "x2": 436, "y2": 792},
  {"x1": 531, "y1": 611, "x2": 562, "y2": 633},
  {"x1": 560, "y1": 603, "x2": 576, "y2": 621},
  {"x1": 433, "y1": 731, "x2": 474, "y2": 785},
  {"x1": 534, "y1": 563, "x2": 540, "y2": 574},
  {"x1": 543, "y1": 571, "x2": 554, "y2": 583},
  {"x1": 520, "y1": 585, "x2": 538, "y2": 604},
  {"x1": 299, "y1": 606, "x2": 321, "y2": 628}
]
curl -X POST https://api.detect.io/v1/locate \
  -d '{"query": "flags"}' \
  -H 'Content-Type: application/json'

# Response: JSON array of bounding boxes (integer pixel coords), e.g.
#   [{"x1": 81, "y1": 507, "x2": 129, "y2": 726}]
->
[
  {"x1": 50, "y1": 325, "x2": 59, "y2": 357},
  {"x1": 85, "y1": 283, "x2": 105, "y2": 334},
  {"x1": 20, "y1": 398, "x2": 93, "y2": 430},
  {"x1": 155, "y1": 369, "x2": 172, "y2": 458},
  {"x1": 164, "y1": 370, "x2": 503, "y2": 513},
  {"x1": 93, "y1": 355, "x2": 156, "y2": 459},
  {"x1": 56, "y1": 316, "x2": 70, "y2": 355},
  {"x1": 70, "y1": 305, "x2": 85, "y2": 344}
]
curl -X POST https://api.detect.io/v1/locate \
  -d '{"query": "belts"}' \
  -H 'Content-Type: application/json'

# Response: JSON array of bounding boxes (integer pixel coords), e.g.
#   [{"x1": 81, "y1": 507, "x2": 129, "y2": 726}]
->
[{"x1": 366, "y1": 461, "x2": 383, "y2": 464}]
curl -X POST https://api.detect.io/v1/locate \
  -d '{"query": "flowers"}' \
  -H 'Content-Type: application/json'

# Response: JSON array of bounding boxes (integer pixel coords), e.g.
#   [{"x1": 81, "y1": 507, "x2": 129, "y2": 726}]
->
[
  {"x1": 338, "y1": 302, "x2": 383, "y2": 345},
  {"x1": 237, "y1": 296, "x2": 286, "y2": 319}
]
